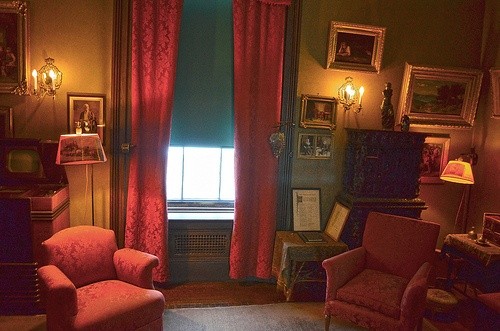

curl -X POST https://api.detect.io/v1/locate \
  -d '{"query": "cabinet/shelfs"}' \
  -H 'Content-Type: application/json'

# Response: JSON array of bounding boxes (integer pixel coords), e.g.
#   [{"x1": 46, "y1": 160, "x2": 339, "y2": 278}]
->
[
  {"x1": 0, "y1": 137, "x2": 70, "y2": 315},
  {"x1": 336, "y1": 128, "x2": 429, "y2": 250}
]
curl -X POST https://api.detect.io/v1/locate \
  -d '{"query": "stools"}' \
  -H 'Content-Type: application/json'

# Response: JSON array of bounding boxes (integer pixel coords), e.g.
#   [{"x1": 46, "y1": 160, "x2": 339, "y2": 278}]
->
[{"x1": 424, "y1": 289, "x2": 457, "y2": 323}]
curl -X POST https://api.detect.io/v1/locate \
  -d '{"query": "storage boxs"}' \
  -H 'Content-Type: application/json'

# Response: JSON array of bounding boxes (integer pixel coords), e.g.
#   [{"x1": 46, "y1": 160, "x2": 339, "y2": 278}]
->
[{"x1": 483, "y1": 213, "x2": 500, "y2": 249}]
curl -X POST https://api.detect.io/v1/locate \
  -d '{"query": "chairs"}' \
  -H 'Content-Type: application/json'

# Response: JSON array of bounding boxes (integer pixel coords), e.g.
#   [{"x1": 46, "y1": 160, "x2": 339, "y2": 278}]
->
[{"x1": 322, "y1": 212, "x2": 440, "y2": 331}]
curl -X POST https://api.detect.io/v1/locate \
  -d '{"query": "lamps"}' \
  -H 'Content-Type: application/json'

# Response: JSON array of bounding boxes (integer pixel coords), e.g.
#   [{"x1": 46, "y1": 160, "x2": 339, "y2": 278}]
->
[
  {"x1": 440, "y1": 148, "x2": 478, "y2": 234},
  {"x1": 32, "y1": 57, "x2": 62, "y2": 105},
  {"x1": 338, "y1": 76, "x2": 365, "y2": 115},
  {"x1": 56, "y1": 121, "x2": 107, "y2": 226}
]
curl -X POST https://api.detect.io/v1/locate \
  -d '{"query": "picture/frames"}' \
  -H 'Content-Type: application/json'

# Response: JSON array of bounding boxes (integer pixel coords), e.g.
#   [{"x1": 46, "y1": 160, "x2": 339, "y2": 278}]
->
[
  {"x1": 326, "y1": 20, "x2": 386, "y2": 75},
  {"x1": 0, "y1": 0, "x2": 31, "y2": 96},
  {"x1": 489, "y1": 67, "x2": 500, "y2": 119},
  {"x1": 0, "y1": 107, "x2": 13, "y2": 138},
  {"x1": 419, "y1": 137, "x2": 450, "y2": 184},
  {"x1": 395, "y1": 61, "x2": 484, "y2": 129},
  {"x1": 297, "y1": 132, "x2": 335, "y2": 161},
  {"x1": 300, "y1": 94, "x2": 339, "y2": 131},
  {"x1": 67, "y1": 92, "x2": 106, "y2": 146}
]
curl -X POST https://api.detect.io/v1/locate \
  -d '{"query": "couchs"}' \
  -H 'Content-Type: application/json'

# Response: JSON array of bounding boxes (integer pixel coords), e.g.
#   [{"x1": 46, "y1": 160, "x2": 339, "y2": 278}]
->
[{"x1": 38, "y1": 225, "x2": 166, "y2": 331}]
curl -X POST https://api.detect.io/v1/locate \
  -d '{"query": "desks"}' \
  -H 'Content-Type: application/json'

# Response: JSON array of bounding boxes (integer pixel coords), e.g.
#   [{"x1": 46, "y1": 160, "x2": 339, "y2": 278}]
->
[
  {"x1": 439, "y1": 234, "x2": 500, "y2": 296},
  {"x1": 272, "y1": 231, "x2": 348, "y2": 301}
]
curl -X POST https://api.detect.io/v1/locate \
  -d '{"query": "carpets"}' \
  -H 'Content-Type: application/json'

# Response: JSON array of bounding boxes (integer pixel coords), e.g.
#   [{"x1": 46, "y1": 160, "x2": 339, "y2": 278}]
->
[{"x1": 162, "y1": 302, "x2": 365, "y2": 331}]
